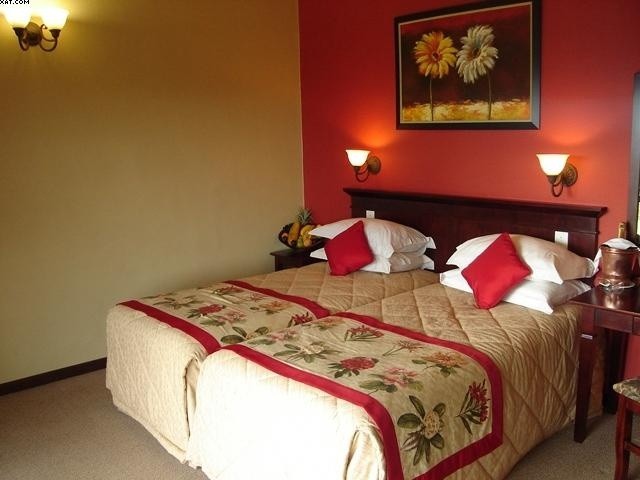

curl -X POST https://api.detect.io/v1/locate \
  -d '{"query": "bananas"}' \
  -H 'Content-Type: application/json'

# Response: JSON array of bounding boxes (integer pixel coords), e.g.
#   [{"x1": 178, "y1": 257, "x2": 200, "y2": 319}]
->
[{"x1": 288, "y1": 221, "x2": 300, "y2": 246}]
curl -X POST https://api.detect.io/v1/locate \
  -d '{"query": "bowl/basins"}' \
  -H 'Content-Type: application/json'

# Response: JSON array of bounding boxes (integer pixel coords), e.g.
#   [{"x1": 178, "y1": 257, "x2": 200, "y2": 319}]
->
[{"x1": 278, "y1": 222, "x2": 323, "y2": 249}]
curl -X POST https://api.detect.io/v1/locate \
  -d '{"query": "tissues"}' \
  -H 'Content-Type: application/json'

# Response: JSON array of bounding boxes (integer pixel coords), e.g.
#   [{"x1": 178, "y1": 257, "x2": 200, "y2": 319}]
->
[{"x1": 593, "y1": 236, "x2": 638, "y2": 289}]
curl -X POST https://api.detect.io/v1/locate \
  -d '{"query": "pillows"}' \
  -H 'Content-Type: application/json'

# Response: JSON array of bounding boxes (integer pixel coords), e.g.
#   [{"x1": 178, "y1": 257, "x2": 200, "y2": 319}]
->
[
  {"x1": 437, "y1": 267, "x2": 591, "y2": 316},
  {"x1": 449, "y1": 233, "x2": 596, "y2": 284},
  {"x1": 308, "y1": 215, "x2": 437, "y2": 257},
  {"x1": 463, "y1": 233, "x2": 532, "y2": 310},
  {"x1": 310, "y1": 248, "x2": 437, "y2": 275},
  {"x1": 323, "y1": 220, "x2": 376, "y2": 276}
]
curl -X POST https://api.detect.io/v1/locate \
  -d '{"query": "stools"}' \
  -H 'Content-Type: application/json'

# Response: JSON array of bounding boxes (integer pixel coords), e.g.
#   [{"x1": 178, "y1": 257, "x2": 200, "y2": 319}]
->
[{"x1": 608, "y1": 377, "x2": 639, "y2": 479}]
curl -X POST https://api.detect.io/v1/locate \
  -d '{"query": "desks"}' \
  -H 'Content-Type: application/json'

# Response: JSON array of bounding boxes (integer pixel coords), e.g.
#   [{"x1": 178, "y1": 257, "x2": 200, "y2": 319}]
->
[{"x1": 568, "y1": 279, "x2": 640, "y2": 444}]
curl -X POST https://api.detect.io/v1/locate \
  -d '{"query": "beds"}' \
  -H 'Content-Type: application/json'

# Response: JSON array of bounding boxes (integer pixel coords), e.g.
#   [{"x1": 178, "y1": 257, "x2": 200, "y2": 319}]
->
[{"x1": 103, "y1": 186, "x2": 606, "y2": 479}]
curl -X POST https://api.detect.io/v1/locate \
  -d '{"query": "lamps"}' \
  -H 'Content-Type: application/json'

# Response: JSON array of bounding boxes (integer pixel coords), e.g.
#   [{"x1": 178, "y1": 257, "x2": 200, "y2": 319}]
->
[
  {"x1": 5, "y1": 3, "x2": 70, "y2": 52},
  {"x1": 345, "y1": 149, "x2": 382, "y2": 183},
  {"x1": 534, "y1": 153, "x2": 579, "y2": 198}
]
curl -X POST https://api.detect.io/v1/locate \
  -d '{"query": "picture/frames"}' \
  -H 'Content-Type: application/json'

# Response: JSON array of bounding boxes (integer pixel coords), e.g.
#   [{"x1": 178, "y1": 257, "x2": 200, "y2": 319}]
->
[{"x1": 394, "y1": 1, "x2": 542, "y2": 131}]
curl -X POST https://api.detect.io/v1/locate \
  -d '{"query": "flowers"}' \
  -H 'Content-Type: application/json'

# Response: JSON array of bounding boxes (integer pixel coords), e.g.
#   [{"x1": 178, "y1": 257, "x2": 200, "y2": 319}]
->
[{"x1": 413, "y1": 23, "x2": 500, "y2": 122}]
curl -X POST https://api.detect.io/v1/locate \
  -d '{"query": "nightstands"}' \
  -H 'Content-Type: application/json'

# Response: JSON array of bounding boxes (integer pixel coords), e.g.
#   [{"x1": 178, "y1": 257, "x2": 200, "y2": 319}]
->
[{"x1": 270, "y1": 249, "x2": 322, "y2": 271}]
